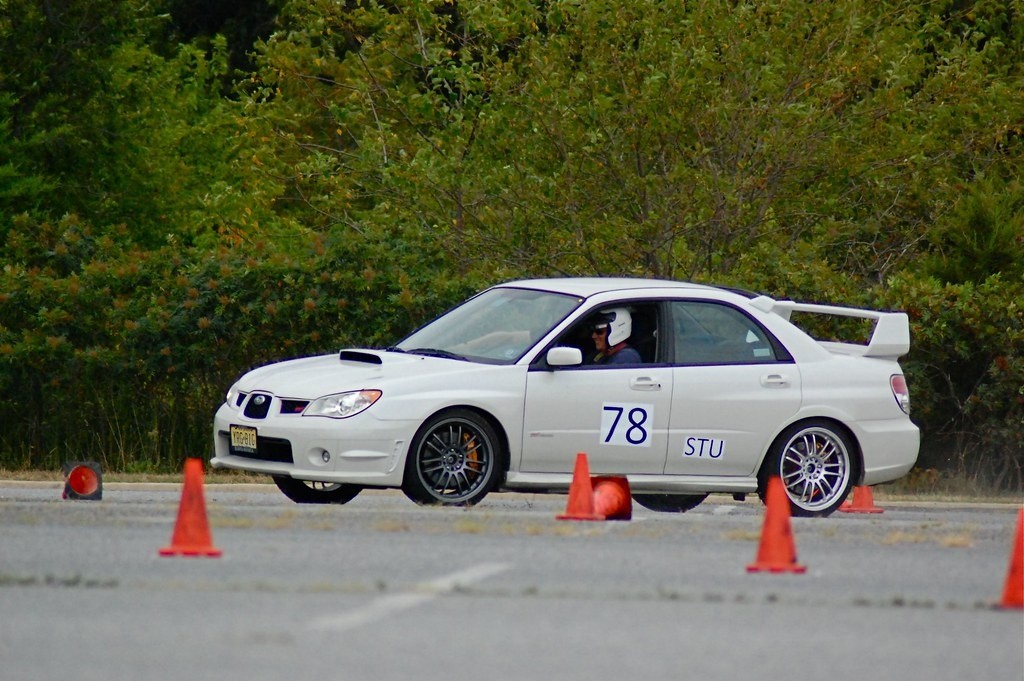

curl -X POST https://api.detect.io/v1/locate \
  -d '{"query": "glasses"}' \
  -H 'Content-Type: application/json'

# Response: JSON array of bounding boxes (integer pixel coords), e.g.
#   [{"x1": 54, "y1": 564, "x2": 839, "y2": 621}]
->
[{"x1": 591, "y1": 329, "x2": 607, "y2": 335}]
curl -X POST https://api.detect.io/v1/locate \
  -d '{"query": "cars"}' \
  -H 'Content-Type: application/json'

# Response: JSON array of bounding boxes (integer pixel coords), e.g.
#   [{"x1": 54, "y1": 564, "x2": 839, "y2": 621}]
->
[{"x1": 208, "y1": 277, "x2": 921, "y2": 517}]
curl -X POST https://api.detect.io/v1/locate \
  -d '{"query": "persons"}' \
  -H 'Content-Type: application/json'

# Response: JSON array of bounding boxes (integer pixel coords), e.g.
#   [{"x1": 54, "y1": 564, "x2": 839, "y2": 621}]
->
[
  {"x1": 590, "y1": 308, "x2": 643, "y2": 364},
  {"x1": 454, "y1": 300, "x2": 568, "y2": 352}
]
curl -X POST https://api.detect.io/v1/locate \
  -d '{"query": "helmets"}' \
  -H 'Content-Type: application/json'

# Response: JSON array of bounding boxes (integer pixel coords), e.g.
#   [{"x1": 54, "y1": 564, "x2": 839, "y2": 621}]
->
[{"x1": 591, "y1": 307, "x2": 632, "y2": 344}]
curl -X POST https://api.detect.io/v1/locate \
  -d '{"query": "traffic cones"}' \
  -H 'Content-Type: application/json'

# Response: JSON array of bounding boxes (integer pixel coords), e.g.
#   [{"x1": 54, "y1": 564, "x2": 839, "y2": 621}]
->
[
  {"x1": 746, "y1": 477, "x2": 806, "y2": 574},
  {"x1": 159, "y1": 458, "x2": 221, "y2": 556},
  {"x1": 994, "y1": 506, "x2": 1024, "y2": 610},
  {"x1": 838, "y1": 486, "x2": 884, "y2": 513},
  {"x1": 589, "y1": 476, "x2": 632, "y2": 519},
  {"x1": 556, "y1": 453, "x2": 605, "y2": 520},
  {"x1": 62, "y1": 461, "x2": 102, "y2": 500},
  {"x1": 806, "y1": 488, "x2": 849, "y2": 511}
]
